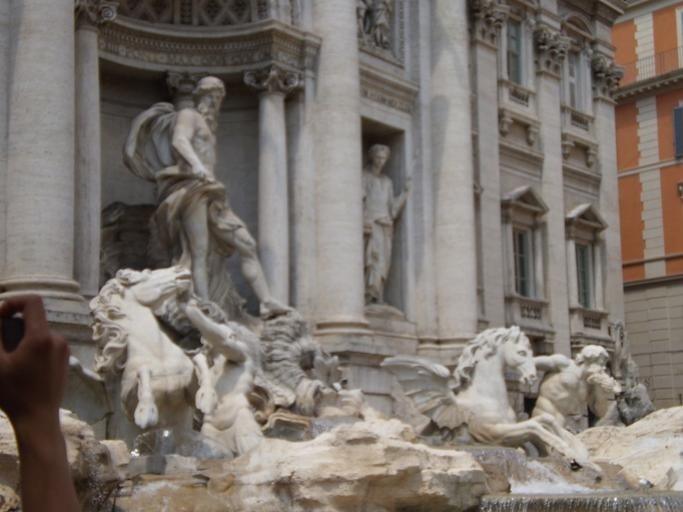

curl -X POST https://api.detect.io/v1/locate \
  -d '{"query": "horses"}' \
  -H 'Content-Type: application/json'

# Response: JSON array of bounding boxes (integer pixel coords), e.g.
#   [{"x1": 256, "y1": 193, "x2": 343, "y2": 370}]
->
[
  {"x1": 63, "y1": 263, "x2": 218, "y2": 454},
  {"x1": 376, "y1": 324, "x2": 604, "y2": 484}
]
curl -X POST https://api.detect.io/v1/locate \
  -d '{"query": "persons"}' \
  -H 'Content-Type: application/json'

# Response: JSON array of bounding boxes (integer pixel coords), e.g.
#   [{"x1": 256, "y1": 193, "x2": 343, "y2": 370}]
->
[
  {"x1": 360, "y1": 140, "x2": 411, "y2": 306},
  {"x1": 172, "y1": 294, "x2": 265, "y2": 462},
  {"x1": 0, "y1": 291, "x2": 87, "y2": 512},
  {"x1": 369, "y1": 0, "x2": 393, "y2": 49},
  {"x1": 530, "y1": 338, "x2": 618, "y2": 435},
  {"x1": 171, "y1": 75, "x2": 296, "y2": 320}
]
